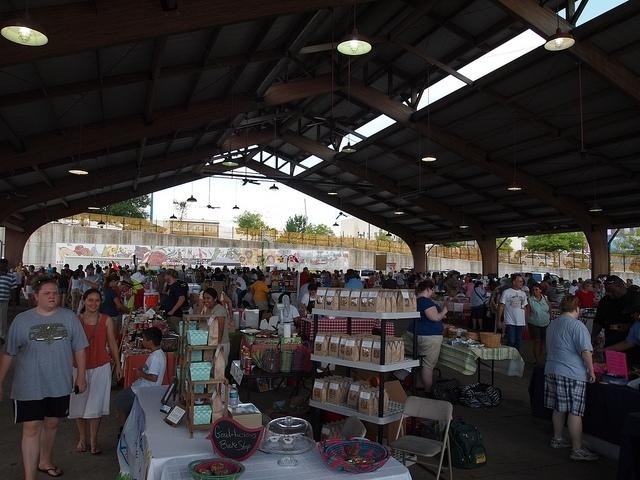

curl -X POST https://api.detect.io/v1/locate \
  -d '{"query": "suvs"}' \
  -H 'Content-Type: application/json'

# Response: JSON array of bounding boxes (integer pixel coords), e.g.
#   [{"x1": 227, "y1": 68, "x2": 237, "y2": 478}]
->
[{"x1": 563, "y1": 252, "x2": 592, "y2": 270}]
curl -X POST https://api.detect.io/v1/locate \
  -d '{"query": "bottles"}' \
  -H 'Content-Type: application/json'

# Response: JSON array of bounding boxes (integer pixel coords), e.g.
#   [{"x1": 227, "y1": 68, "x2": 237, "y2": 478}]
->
[
  {"x1": 229, "y1": 383, "x2": 239, "y2": 407},
  {"x1": 123, "y1": 306, "x2": 165, "y2": 348},
  {"x1": 233, "y1": 312, "x2": 240, "y2": 329}
]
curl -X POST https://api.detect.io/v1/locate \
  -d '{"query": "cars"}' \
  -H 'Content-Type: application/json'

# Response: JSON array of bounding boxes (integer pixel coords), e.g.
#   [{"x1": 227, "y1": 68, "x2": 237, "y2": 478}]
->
[{"x1": 519, "y1": 253, "x2": 560, "y2": 268}]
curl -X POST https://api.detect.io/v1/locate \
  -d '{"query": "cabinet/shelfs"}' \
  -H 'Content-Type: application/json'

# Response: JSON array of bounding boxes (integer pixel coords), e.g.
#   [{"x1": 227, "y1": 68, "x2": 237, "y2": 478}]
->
[
  {"x1": 175, "y1": 314, "x2": 229, "y2": 439},
  {"x1": 308, "y1": 308, "x2": 421, "y2": 467}
]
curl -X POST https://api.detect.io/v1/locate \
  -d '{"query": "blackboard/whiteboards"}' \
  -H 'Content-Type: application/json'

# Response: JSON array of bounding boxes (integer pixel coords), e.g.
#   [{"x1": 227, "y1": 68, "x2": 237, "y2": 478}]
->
[{"x1": 208, "y1": 416, "x2": 264, "y2": 462}]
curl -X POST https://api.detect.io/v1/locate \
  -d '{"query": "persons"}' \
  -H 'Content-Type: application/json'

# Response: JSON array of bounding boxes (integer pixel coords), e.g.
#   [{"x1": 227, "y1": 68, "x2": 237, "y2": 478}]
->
[{"x1": 0, "y1": 258, "x2": 640, "y2": 480}]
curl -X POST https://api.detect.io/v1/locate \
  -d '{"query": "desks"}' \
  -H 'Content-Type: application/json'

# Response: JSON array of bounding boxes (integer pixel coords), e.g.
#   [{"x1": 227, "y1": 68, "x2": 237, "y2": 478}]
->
[
  {"x1": 116, "y1": 385, "x2": 242, "y2": 480},
  {"x1": 239, "y1": 333, "x2": 312, "y2": 374},
  {"x1": 161, "y1": 442, "x2": 412, "y2": 480},
  {"x1": 528, "y1": 363, "x2": 640, "y2": 461},
  {"x1": 117, "y1": 315, "x2": 176, "y2": 390},
  {"x1": 437, "y1": 337, "x2": 525, "y2": 386}
]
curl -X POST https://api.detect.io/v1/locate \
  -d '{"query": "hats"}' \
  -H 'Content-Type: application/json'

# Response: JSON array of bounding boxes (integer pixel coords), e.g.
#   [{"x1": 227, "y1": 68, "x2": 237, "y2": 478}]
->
[{"x1": 119, "y1": 277, "x2": 135, "y2": 289}]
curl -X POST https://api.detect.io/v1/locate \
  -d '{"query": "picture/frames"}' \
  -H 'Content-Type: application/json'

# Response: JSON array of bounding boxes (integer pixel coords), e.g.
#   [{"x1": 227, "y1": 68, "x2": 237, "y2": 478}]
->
[
  {"x1": 163, "y1": 404, "x2": 188, "y2": 428},
  {"x1": 161, "y1": 381, "x2": 175, "y2": 404}
]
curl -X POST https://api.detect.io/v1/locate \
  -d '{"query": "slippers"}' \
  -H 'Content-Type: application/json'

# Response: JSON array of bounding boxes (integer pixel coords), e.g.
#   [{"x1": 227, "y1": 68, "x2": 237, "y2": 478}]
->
[
  {"x1": 38, "y1": 465, "x2": 64, "y2": 477},
  {"x1": 77, "y1": 442, "x2": 101, "y2": 455}
]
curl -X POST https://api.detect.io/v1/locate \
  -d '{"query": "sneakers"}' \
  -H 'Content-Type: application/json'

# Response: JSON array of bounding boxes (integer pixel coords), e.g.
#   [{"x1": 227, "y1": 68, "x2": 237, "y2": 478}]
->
[
  {"x1": 550, "y1": 435, "x2": 573, "y2": 449},
  {"x1": 570, "y1": 447, "x2": 599, "y2": 462}
]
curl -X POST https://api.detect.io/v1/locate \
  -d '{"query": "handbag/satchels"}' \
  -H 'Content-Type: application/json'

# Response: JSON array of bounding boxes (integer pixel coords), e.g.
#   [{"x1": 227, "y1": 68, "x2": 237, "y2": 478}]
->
[
  {"x1": 483, "y1": 300, "x2": 491, "y2": 311},
  {"x1": 520, "y1": 327, "x2": 531, "y2": 341},
  {"x1": 462, "y1": 381, "x2": 502, "y2": 410},
  {"x1": 434, "y1": 378, "x2": 460, "y2": 405}
]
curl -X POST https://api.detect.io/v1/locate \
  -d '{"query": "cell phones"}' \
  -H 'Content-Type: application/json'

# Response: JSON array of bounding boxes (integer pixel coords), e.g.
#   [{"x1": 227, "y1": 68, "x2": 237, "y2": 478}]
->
[{"x1": 74, "y1": 385, "x2": 79, "y2": 394}]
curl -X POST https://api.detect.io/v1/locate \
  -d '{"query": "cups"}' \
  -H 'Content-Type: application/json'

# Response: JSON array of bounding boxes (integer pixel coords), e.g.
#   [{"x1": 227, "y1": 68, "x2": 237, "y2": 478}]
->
[{"x1": 283, "y1": 321, "x2": 292, "y2": 338}]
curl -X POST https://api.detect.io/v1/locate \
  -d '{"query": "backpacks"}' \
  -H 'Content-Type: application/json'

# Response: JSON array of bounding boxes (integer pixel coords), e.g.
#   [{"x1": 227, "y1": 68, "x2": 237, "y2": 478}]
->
[{"x1": 443, "y1": 418, "x2": 487, "y2": 470}]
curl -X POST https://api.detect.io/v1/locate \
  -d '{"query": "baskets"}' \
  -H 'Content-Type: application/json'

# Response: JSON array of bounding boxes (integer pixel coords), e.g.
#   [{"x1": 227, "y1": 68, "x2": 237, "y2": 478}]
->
[
  {"x1": 443, "y1": 326, "x2": 479, "y2": 342},
  {"x1": 479, "y1": 330, "x2": 502, "y2": 348}
]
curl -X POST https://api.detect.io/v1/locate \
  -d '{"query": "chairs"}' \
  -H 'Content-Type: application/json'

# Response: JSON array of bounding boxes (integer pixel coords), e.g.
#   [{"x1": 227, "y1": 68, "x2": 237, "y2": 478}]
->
[{"x1": 390, "y1": 396, "x2": 454, "y2": 480}]
